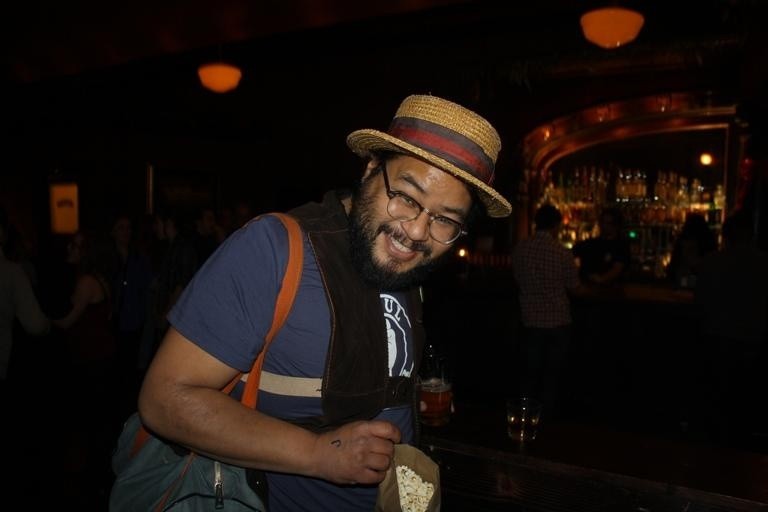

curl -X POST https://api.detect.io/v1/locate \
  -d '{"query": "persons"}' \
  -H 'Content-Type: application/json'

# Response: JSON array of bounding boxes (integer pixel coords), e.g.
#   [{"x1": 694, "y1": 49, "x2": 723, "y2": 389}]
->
[
  {"x1": 518, "y1": 207, "x2": 598, "y2": 416},
  {"x1": 673, "y1": 233, "x2": 699, "y2": 274},
  {"x1": 572, "y1": 208, "x2": 628, "y2": 290},
  {"x1": 690, "y1": 208, "x2": 768, "y2": 451},
  {"x1": 137, "y1": 145, "x2": 484, "y2": 512},
  {"x1": 1, "y1": 200, "x2": 254, "y2": 466}
]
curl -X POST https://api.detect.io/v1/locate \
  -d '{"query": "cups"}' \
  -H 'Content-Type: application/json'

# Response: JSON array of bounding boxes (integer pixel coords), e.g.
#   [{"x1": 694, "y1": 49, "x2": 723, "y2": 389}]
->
[
  {"x1": 505, "y1": 396, "x2": 538, "y2": 441},
  {"x1": 418, "y1": 377, "x2": 452, "y2": 427}
]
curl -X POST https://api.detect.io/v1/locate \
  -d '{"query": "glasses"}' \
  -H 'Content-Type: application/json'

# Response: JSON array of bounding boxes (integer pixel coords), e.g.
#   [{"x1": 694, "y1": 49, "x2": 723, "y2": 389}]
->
[{"x1": 380, "y1": 158, "x2": 466, "y2": 246}]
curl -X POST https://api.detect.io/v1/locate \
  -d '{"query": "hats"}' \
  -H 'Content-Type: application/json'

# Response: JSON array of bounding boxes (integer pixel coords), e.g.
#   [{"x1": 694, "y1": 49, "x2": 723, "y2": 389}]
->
[{"x1": 345, "y1": 92, "x2": 514, "y2": 219}]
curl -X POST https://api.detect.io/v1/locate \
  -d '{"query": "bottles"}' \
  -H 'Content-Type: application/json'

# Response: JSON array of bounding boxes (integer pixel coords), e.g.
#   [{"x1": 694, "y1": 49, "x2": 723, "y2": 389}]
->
[
  {"x1": 555, "y1": 165, "x2": 701, "y2": 205},
  {"x1": 714, "y1": 184, "x2": 725, "y2": 209}
]
